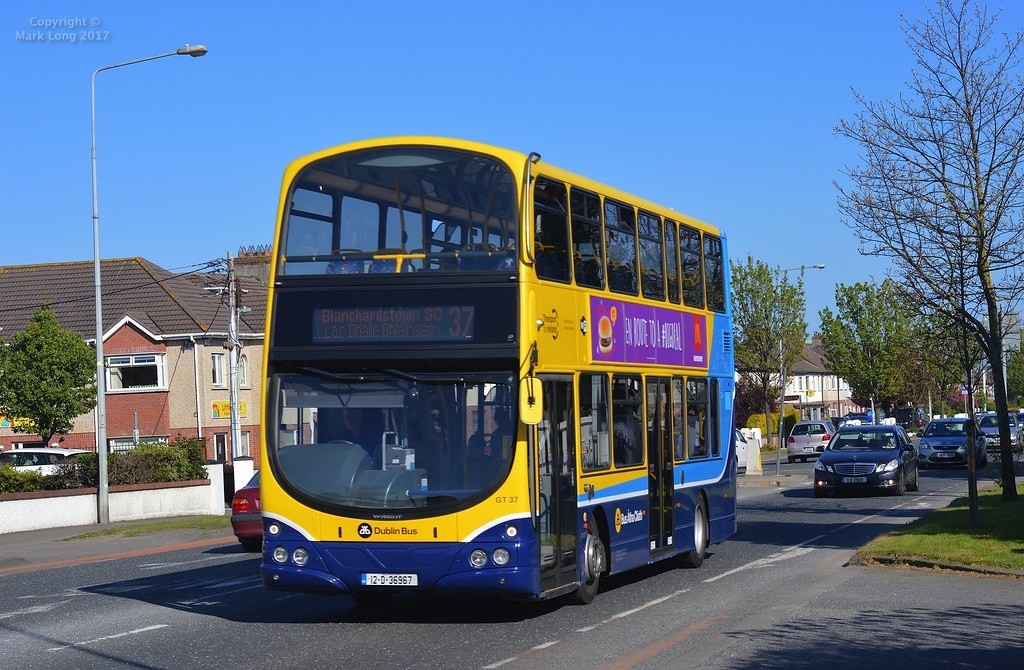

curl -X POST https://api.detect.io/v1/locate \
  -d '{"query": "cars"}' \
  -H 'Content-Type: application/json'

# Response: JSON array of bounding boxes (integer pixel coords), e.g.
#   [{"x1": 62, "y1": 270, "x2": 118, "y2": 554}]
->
[
  {"x1": 831, "y1": 412, "x2": 874, "y2": 432},
  {"x1": 918, "y1": 418, "x2": 988, "y2": 469},
  {"x1": 230, "y1": 469, "x2": 261, "y2": 554},
  {"x1": 814, "y1": 425, "x2": 921, "y2": 499},
  {"x1": 978, "y1": 413, "x2": 1024, "y2": 453},
  {"x1": 733, "y1": 428, "x2": 748, "y2": 474},
  {"x1": 1015, "y1": 412, "x2": 1024, "y2": 432}
]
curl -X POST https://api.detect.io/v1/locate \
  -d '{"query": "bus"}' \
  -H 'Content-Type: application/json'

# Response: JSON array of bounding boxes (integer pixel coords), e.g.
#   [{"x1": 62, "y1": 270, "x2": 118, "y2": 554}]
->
[{"x1": 258, "y1": 137, "x2": 738, "y2": 607}]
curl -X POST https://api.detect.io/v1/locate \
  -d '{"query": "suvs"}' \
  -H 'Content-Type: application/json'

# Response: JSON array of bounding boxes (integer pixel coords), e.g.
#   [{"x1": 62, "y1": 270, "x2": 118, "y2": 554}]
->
[
  {"x1": 0, "y1": 448, "x2": 94, "y2": 480},
  {"x1": 786, "y1": 420, "x2": 838, "y2": 464}
]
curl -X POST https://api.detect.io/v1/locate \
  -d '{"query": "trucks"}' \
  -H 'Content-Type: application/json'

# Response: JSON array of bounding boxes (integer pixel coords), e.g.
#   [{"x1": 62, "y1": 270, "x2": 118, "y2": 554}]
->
[{"x1": 894, "y1": 406, "x2": 931, "y2": 426}]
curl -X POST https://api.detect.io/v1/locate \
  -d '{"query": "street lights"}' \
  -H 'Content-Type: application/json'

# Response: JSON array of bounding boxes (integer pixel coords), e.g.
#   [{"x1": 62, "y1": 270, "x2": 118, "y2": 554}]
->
[
  {"x1": 778, "y1": 262, "x2": 827, "y2": 387},
  {"x1": 90, "y1": 42, "x2": 209, "y2": 525}
]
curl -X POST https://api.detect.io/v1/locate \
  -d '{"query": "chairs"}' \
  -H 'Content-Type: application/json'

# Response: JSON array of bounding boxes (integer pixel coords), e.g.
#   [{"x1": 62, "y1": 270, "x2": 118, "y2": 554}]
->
[{"x1": 327, "y1": 240, "x2": 700, "y2": 308}]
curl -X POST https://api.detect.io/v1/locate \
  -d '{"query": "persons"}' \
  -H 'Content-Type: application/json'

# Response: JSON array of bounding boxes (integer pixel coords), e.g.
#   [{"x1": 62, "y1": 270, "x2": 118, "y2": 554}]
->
[
  {"x1": 916, "y1": 419, "x2": 924, "y2": 435},
  {"x1": 329, "y1": 408, "x2": 376, "y2": 450},
  {"x1": 489, "y1": 408, "x2": 514, "y2": 456},
  {"x1": 431, "y1": 409, "x2": 448, "y2": 451},
  {"x1": 675, "y1": 403, "x2": 706, "y2": 459}
]
